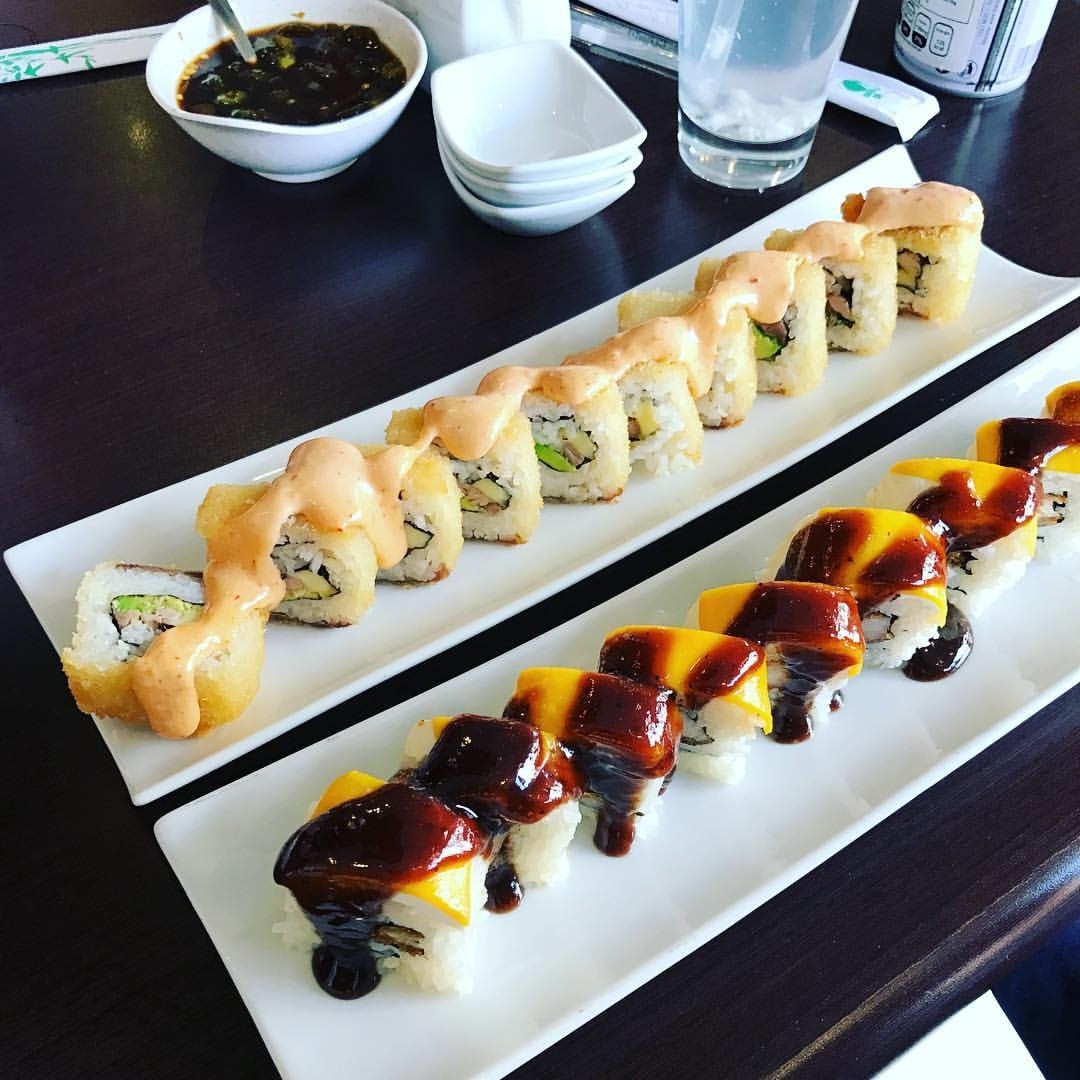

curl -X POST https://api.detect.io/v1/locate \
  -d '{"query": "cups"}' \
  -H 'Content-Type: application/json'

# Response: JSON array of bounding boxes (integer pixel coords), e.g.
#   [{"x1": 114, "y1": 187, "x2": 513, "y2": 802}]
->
[{"x1": 673, "y1": 1, "x2": 858, "y2": 192}]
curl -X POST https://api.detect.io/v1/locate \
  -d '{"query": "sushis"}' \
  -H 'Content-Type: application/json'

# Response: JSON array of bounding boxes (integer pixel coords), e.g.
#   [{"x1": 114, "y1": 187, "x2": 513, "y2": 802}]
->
[
  {"x1": 197, "y1": 473, "x2": 381, "y2": 631},
  {"x1": 1031, "y1": 378, "x2": 1080, "y2": 424},
  {"x1": 493, "y1": 663, "x2": 684, "y2": 849},
  {"x1": 758, "y1": 504, "x2": 952, "y2": 675},
  {"x1": 841, "y1": 177, "x2": 983, "y2": 323},
  {"x1": 266, "y1": 766, "x2": 493, "y2": 1006},
  {"x1": 385, "y1": 390, "x2": 544, "y2": 546},
  {"x1": 962, "y1": 417, "x2": 1080, "y2": 526},
  {"x1": 56, "y1": 537, "x2": 269, "y2": 742},
  {"x1": 593, "y1": 618, "x2": 778, "y2": 792},
  {"x1": 684, "y1": 578, "x2": 867, "y2": 751},
  {"x1": 856, "y1": 453, "x2": 1047, "y2": 617},
  {"x1": 696, "y1": 250, "x2": 826, "y2": 398},
  {"x1": 395, "y1": 715, "x2": 581, "y2": 905},
  {"x1": 286, "y1": 429, "x2": 465, "y2": 588},
  {"x1": 614, "y1": 280, "x2": 753, "y2": 431},
  {"x1": 764, "y1": 217, "x2": 897, "y2": 362},
  {"x1": 555, "y1": 318, "x2": 709, "y2": 480},
  {"x1": 475, "y1": 356, "x2": 633, "y2": 513}
]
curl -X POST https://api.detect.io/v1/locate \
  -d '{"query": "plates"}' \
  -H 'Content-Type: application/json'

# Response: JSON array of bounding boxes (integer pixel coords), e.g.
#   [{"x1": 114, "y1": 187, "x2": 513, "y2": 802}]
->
[
  {"x1": 429, "y1": 36, "x2": 647, "y2": 237},
  {"x1": 1, "y1": 145, "x2": 1080, "y2": 1080}
]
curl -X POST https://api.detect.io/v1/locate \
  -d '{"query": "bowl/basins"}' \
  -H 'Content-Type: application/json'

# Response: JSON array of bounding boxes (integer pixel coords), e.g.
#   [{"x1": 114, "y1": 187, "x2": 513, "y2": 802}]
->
[{"x1": 145, "y1": 0, "x2": 429, "y2": 186}]
